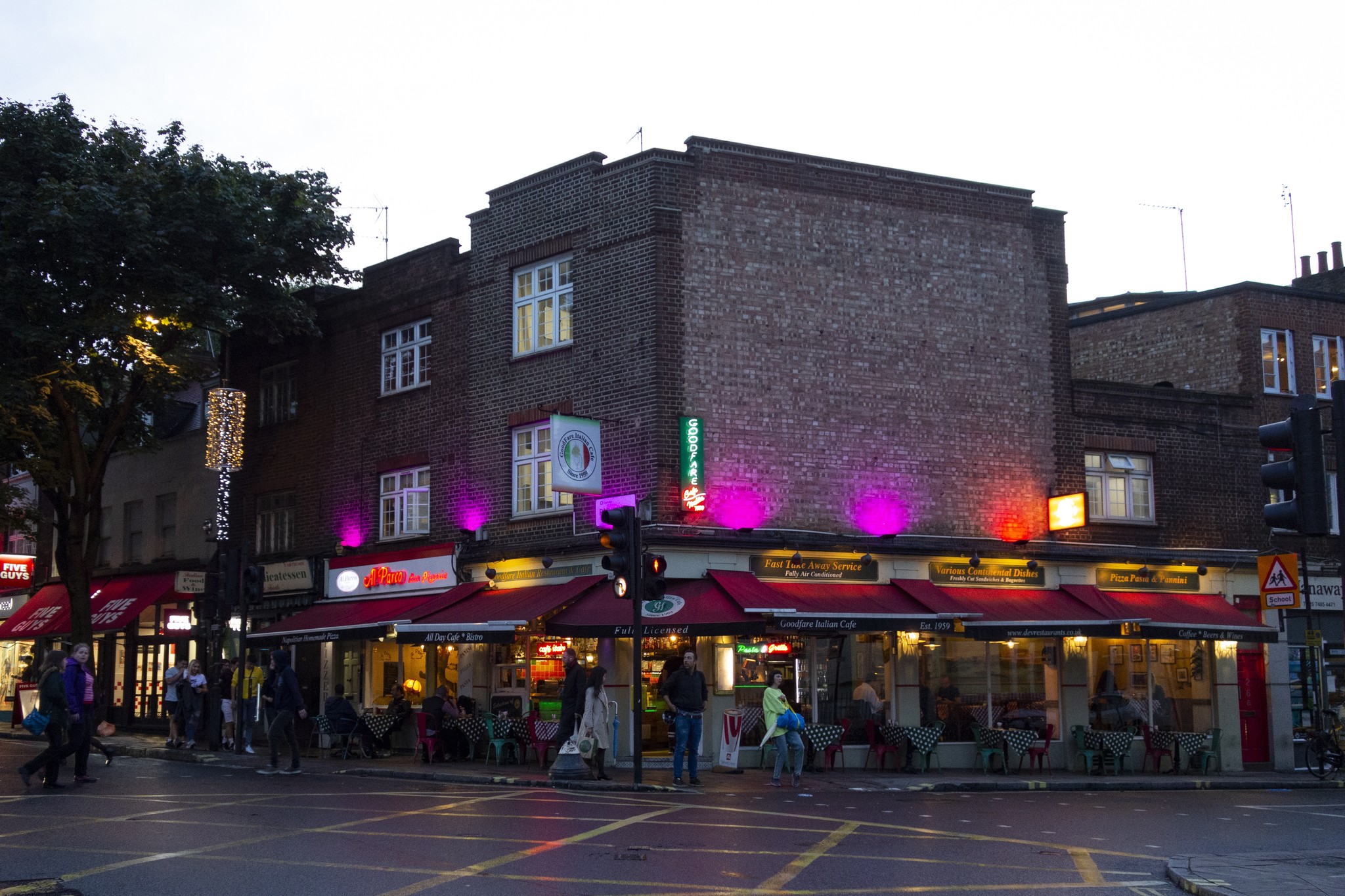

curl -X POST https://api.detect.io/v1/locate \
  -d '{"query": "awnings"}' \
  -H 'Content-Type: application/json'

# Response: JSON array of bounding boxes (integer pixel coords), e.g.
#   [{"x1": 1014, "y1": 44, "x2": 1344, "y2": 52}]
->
[
  {"x1": 0, "y1": 573, "x2": 176, "y2": 641},
  {"x1": 247, "y1": 568, "x2": 1279, "y2": 643}
]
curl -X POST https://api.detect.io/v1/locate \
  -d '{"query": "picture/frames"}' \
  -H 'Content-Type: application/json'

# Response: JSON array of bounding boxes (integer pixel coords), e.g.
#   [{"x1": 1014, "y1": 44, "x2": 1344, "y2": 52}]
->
[{"x1": 1109, "y1": 644, "x2": 1189, "y2": 688}]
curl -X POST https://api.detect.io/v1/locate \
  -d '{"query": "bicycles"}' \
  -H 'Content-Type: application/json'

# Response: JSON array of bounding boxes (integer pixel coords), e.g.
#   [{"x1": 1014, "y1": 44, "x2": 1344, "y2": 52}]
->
[{"x1": 1305, "y1": 708, "x2": 1345, "y2": 777}]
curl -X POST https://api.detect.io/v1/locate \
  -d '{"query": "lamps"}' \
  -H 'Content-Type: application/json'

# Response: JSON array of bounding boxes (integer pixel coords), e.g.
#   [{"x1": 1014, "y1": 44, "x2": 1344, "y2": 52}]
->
[
  {"x1": 1023, "y1": 551, "x2": 1037, "y2": 572},
  {"x1": 542, "y1": 546, "x2": 565, "y2": 568},
  {"x1": 1181, "y1": 562, "x2": 1207, "y2": 575},
  {"x1": 741, "y1": 659, "x2": 769, "y2": 672},
  {"x1": 485, "y1": 558, "x2": 506, "y2": 579},
  {"x1": 961, "y1": 548, "x2": 980, "y2": 567},
  {"x1": 1127, "y1": 558, "x2": 1148, "y2": 577},
  {"x1": 853, "y1": 544, "x2": 872, "y2": 566},
  {"x1": 784, "y1": 543, "x2": 802, "y2": 565}
]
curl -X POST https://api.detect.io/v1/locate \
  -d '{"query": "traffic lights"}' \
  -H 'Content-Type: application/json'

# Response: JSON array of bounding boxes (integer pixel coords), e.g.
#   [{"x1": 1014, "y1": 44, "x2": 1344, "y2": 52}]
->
[
  {"x1": 641, "y1": 553, "x2": 667, "y2": 600},
  {"x1": 597, "y1": 505, "x2": 633, "y2": 599},
  {"x1": 245, "y1": 565, "x2": 264, "y2": 605},
  {"x1": 1258, "y1": 412, "x2": 1327, "y2": 535},
  {"x1": 214, "y1": 551, "x2": 235, "y2": 621}
]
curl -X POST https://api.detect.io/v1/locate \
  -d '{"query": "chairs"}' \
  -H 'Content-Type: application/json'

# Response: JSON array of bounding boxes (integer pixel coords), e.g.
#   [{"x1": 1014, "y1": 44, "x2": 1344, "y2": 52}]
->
[
  {"x1": 757, "y1": 716, "x2": 945, "y2": 774},
  {"x1": 971, "y1": 723, "x2": 1223, "y2": 777},
  {"x1": 307, "y1": 712, "x2": 559, "y2": 772}
]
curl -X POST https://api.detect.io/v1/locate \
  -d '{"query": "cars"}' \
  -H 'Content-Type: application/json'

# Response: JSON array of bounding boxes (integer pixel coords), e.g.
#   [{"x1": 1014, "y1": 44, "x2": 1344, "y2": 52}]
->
[{"x1": 1089, "y1": 692, "x2": 1140, "y2": 727}]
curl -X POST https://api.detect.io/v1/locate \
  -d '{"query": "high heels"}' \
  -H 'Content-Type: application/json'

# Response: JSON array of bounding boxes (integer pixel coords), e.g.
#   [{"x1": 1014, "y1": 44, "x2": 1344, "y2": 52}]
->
[{"x1": 597, "y1": 772, "x2": 613, "y2": 781}]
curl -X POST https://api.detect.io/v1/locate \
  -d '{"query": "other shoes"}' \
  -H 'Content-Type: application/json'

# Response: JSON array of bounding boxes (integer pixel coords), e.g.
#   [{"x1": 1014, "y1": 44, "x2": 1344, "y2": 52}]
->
[
  {"x1": 791, "y1": 774, "x2": 800, "y2": 787},
  {"x1": 166, "y1": 738, "x2": 185, "y2": 748},
  {"x1": 245, "y1": 746, "x2": 255, "y2": 754},
  {"x1": 105, "y1": 751, "x2": 115, "y2": 764},
  {"x1": 257, "y1": 764, "x2": 279, "y2": 773},
  {"x1": 280, "y1": 766, "x2": 301, "y2": 774},
  {"x1": 19, "y1": 765, "x2": 33, "y2": 786},
  {"x1": 771, "y1": 778, "x2": 781, "y2": 786},
  {"x1": 689, "y1": 777, "x2": 704, "y2": 787},
  {"x1": 422, "y1": 754, "x2": 429, "y2": 763},
  {"x1": 362, "y1": 747, "x2": 372, "y2": 758},
  {"x1": 43, "y1": 779, "x2": 66, "y2": 789},
  {"x1": 375, "y1": 749, "x2": 391, "y2": 757},
  {"x1": 220, "y1": 741, "x2": 235, "y2": 751},
  {"x1": 74, "y1": 774, "x2": 97, "y2": 782},
  {"x1": 672, "y1": 777, "x2": 688, "y2": 787},
  {"x1": 186, "y1": 739, "x2": 196, "y2": 750}
]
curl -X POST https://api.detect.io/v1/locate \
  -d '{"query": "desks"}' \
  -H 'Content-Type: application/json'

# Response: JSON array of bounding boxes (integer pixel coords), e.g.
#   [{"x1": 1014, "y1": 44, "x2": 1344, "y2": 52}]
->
[
  {"x1": 798, "y1": 723, "x2": 845, "y2": 772},
  {"x1": 317, "y1": 715, "x2": 400, "y2": 755},
  {"x1": 442, "y1": 718, "x2": 532, "y2": 761},
  {"x1": 979, "y1": 728, "x2": 1038, "y2": 773},
  {"x1": 1151, "y1": 730, "x2": 1207, "y2": 775},
  {"x1": 879, "y1": 726, "x2": 942, "y2": 772},
  {"x1": 1084, "y1": 730, "x2": 1134, "y2": 776}
]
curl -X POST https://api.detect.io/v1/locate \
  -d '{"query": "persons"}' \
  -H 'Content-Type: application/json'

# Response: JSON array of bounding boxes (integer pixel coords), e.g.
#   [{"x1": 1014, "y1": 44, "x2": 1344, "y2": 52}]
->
[
  {"x1": 1091, "y1": 670, "x2": 1127, "y2": 731},
  {"x1": 763, "y1": 670, "x2": 805, "y2": 788},
  {"x1": 324, "y1": 685, "x2": 373, "y2": 759},
  {"x1": 380, "y1": 683, "x2": 411, "y2": 757},
  {"x1": 17, "y1": 645, "x2": 113, "y2": 791},
  {"x1": 165, "y1": 649, "x2": 306, "y2": 775},
  {"x1": 421, "y1": 684, "x2": 459, "y2": 765},
  {"x1": 0, "y1": 650, "x2": 11, "y2": 705},
  {"x1": 742, "y1": 668, "x2": 751, "y2": 683},
  {"x1": 555, "y1": 648, "x2": 614, "y2": 781},
  {"x1": 1146, "y1": 674, "x2": 1166, "y2": 732},
  {"x1": 919, "y1": 675, "x2": 961, "y2": 742},
  {"x1": 661, "y1": 652, "x2": 708, "y2": 786},
  {"x1": 852, "y1": 672, "x2": 886, "y2": 722},
  {"x1": 658, "y1": 642, "x2": 690, "y2": 755}
]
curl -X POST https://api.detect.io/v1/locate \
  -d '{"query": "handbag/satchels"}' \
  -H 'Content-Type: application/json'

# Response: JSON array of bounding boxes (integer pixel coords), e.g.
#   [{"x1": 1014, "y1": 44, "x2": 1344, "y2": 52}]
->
[
  {"x1": 570, "y1": 716, "x2": 582, "y2": 745},
  {"x1": 777, "y1": 709, "x2": 805, "y2": 731},
  {"x1": 576, "y1": 732, "x2": 599, "y2": 759},
  {"x1": 662, "y1": 711, "x2": 676, "y2": 726},
  {"x1": 22, "y1": 708, "x2": 49, "y2": 736}
]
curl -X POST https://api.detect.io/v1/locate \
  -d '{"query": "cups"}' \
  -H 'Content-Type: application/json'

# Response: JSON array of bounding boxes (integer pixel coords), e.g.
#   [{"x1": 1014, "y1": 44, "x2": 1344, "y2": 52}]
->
[
  {"x1": 376, "y1": 708, "x2": 383, "y2": 715},
  {"x1": 183, "y1": 669, "x2": 188, "y2": 679},
  {"x1": 723, "y1": 709, "x2": 744, "y2": 753}
]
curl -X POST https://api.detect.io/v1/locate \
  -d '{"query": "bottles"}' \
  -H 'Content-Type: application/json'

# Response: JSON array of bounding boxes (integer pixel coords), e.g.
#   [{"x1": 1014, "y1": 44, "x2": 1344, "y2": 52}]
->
[
  {"x1": 478, "y1": 706, "x2": 482, "y2": 718},
  {"x1": 641, "y1": 636, "x2": 690, "y2": 651},
  {"x1": 734, "y1": 635, "x2": 830, "y2": 725}
]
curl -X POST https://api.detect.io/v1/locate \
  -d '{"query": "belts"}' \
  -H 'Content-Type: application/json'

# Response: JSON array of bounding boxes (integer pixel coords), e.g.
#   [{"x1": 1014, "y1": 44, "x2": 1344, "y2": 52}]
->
[{"x1": 678, "y1": 713, "x2": 702, "y2": 719}]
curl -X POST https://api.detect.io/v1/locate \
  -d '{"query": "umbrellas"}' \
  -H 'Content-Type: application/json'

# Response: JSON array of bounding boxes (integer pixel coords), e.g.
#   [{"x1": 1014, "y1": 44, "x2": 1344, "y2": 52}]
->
[{"x1": 609, "y1": 701, "x2": 619, "y2": 765}]
